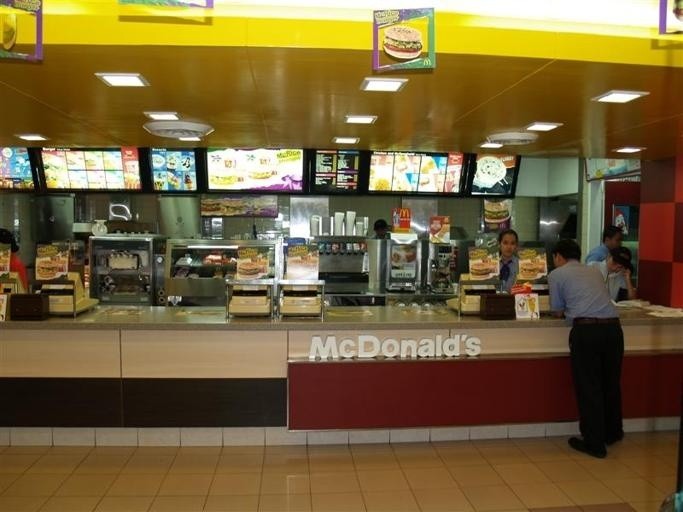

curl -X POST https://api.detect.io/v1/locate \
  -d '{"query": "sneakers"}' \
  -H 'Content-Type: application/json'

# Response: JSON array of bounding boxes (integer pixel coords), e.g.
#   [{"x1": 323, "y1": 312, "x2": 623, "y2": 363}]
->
[{"x1": 568, "y1": 436, "x2": 607, "y2": 459}]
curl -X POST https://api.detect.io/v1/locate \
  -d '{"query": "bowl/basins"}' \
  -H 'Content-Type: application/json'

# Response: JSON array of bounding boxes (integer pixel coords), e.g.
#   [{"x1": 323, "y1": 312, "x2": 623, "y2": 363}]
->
[{"x1": 152, "y1": 154, "x2": 166, "y2": 169}]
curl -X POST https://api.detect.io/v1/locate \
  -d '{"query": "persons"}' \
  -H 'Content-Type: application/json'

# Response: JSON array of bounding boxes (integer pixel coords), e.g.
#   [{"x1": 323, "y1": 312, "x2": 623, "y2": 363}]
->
[
  {"x1": 495, "y1": 228, "x2": 520, "y2": 291},
  {"x1": 546, "y1": 235, "x2": 625, "y2": 459},
  {"x1": 369, "y1": 218, "x2": 388, "y2": 240},
  {"x1": 587, "y1": 246, "x2": 636, "y2": 303},
  {"x1": 0, "y1": 228, "x2": 29, "y2": 290},
  {"x1": 585, "y1": 224, "x2": 623, "y2": 264}
]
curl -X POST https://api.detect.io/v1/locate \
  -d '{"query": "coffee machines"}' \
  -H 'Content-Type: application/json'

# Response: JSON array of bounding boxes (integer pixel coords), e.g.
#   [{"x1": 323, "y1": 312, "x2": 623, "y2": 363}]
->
[{"x1": 425, "y1": 240, "x2": 455, "y2": 294}]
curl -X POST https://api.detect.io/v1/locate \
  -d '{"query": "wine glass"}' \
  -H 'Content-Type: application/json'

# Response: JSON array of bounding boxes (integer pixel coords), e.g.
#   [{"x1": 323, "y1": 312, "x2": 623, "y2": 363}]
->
[
  {"x1": 406, "y1": 172, "x2": 419, "y2": 191},
  {"x1": 445, "y1": 169, "x2": 461, "y2": 192},
  {"x1": 433, "y1": 173, "x2": 445, "y2": 192}
]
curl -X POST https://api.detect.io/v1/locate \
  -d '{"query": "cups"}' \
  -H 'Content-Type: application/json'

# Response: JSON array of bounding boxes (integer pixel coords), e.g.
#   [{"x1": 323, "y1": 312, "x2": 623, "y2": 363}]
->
[
  {"x1": 125, "y1": 161, "x2": 140, "y2": 181},
  {"x1": 396, "y1": 302, "x2": 445, "y2": 313},
  {"x1": 310, "y1": 210, "x2": 364, "y2": 236}
]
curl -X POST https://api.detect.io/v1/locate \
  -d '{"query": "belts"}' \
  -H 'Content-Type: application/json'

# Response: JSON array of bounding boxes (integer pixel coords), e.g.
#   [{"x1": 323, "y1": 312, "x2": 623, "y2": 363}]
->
[{"x1": 575, "y1": 318, "x2": 620, "y2": 325}]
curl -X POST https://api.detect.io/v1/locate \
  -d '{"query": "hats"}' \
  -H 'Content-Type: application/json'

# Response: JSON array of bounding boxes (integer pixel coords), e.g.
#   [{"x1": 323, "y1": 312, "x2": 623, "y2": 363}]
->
[
  {"x1": 612, "y1": 247, "x2": 632, "y2": 267},
  {"x1": 374, "y1": 220, "x2": 387, "y2": 230}
]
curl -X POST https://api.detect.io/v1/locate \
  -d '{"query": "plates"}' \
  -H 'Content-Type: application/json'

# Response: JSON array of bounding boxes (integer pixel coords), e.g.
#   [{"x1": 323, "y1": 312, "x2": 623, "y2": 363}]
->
[{"x1": 478, "y1": 156, "x2": 507, "y2": 182}]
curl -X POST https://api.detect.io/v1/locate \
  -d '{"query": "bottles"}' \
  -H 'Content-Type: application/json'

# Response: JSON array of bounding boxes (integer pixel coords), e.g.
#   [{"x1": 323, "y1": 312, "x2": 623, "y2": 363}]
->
[{"x1": 393, "y1": 212, "x2": 400, "y2": 227}]
